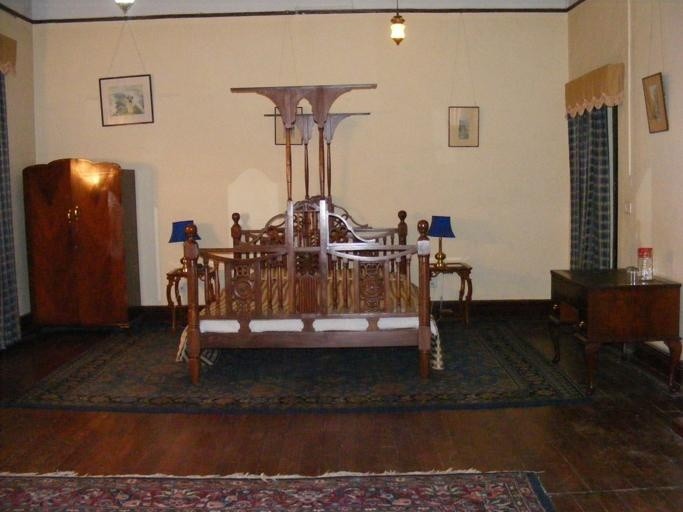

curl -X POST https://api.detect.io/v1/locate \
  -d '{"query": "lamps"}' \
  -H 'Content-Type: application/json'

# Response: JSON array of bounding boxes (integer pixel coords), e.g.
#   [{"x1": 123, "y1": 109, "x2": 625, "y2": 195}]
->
[
  {"x1": 425, "y1": 214, "x2": 456, "y2": 268},
  {"x1": 391, "y1": 1, "x2": 407, "y2": 46},
  {"x1": 168, "y1": 220, "x2": 201, "y2": 270}
]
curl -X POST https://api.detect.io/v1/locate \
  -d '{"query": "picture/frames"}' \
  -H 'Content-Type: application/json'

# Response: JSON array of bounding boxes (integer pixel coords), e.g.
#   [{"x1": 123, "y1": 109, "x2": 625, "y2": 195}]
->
[
  {"x1": 98, "y1": 74, "x2": 154, "y2": 127},
  {"x1": 641, "y1": 72, "x2": 669, "y2": 134},
  {"x1": 447, "y1": 105, "x2": 480, "y2": 148},
  {"x1": 275, "y1": 106, "x2": 304, "y2": 145}
]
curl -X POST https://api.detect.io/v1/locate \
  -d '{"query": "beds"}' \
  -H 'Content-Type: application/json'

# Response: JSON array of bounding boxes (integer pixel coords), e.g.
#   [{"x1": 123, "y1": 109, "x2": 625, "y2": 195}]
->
[{"x1": 184, "y1": 195, "x2": 432, "y2": 387}]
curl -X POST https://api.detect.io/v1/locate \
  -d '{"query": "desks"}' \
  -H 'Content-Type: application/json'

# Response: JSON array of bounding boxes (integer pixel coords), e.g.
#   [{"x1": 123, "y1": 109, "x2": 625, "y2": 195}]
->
[
  {"x1": 428, "y1": 261, "x2": 473, "y2": 327},
  {"x1": 548, "y1": 269, "x2": 681, "y2": 396},
  {"x1": 167, "y1": 268, "x2": 219, "y2": 333}
]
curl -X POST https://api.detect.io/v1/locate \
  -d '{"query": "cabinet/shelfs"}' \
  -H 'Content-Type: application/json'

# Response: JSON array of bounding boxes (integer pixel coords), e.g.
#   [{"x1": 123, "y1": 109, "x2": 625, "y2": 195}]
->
[{"x1": 19, "y1": 157, "x2": 143, "y2": 332}]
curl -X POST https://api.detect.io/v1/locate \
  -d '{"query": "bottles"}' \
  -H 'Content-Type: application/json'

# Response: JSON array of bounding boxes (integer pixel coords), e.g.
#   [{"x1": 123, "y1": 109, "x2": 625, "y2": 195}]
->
[{"x1": 637, "y1": 246, "x2": 654, "y2": 281}]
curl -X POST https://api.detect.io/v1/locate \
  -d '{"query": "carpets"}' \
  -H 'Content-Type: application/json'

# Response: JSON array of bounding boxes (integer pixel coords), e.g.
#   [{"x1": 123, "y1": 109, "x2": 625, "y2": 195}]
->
[
  {"x1": 1, "y1": 466, "x2": 557, "y2": 512},
  {"x1": 8, "y1": 315, "x2": 589, "y2": 409}
]
